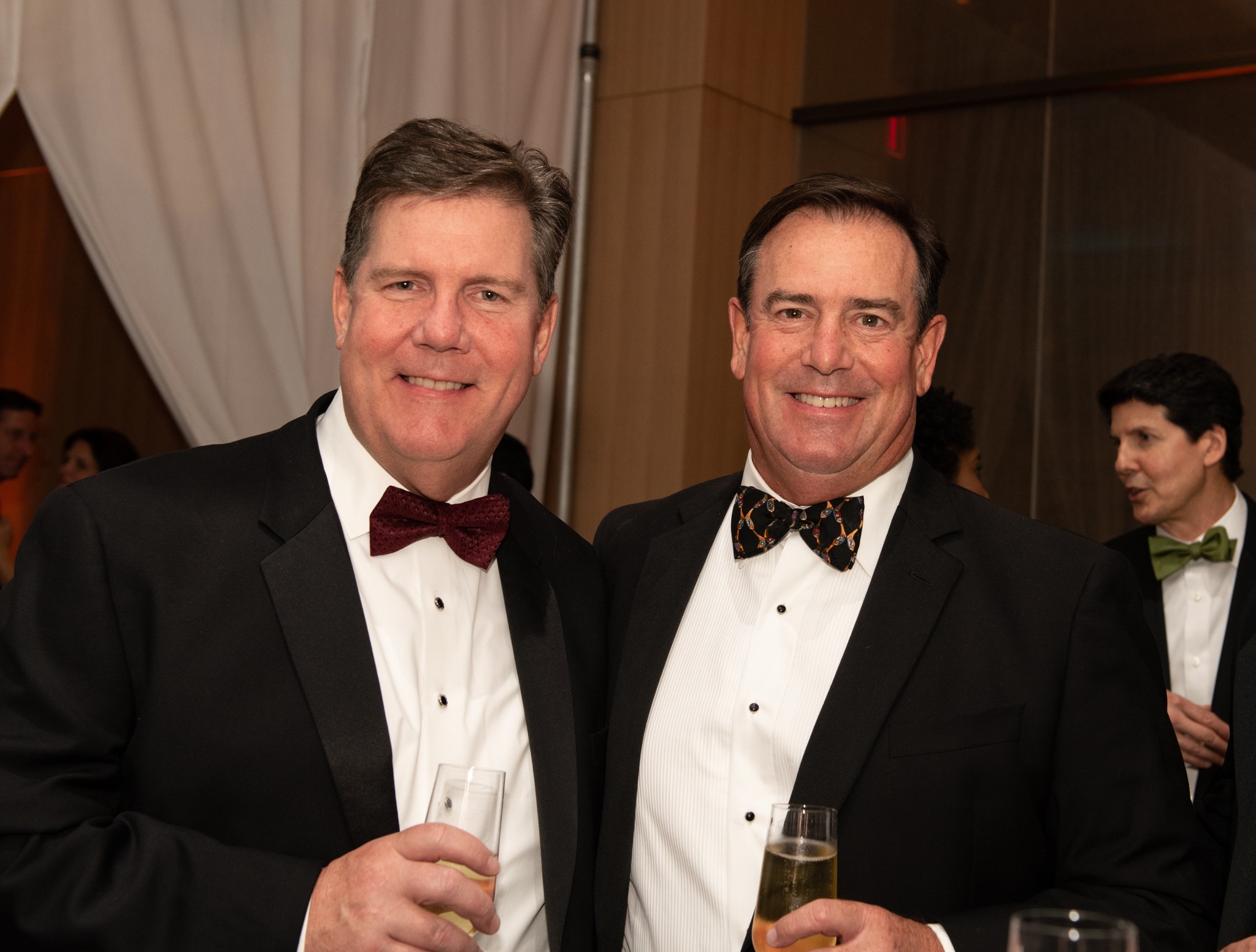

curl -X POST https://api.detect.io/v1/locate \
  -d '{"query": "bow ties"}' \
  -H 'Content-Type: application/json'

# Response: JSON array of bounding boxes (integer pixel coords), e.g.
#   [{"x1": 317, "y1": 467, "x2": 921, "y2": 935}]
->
[
  {"x1": 369, "y1": 485, "x2": 510, "y2": 570},
  {"x1": 731, "y1": 485, "x2": 865, "y2": 572},
  {"x1": 1148, "y1": 526, "x2": 1238, "y2": 582}
]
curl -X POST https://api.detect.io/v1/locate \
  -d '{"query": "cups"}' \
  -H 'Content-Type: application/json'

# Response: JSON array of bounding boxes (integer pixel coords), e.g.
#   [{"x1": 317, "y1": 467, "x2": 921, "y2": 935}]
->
[
  {"x1": 421, "y1": 764, "x2": 505, "y2": 939},
  {"x1": 1008, "y1": 909, "x2": 1138, "y2": 952},
  {"x1": 750, "y1": 804, "x2": 839, "y2": 952}
]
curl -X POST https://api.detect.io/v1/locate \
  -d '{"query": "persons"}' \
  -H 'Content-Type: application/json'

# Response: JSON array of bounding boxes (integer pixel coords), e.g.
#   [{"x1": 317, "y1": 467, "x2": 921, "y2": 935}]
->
[
  {"x1": 912, "y1": 384, "x2": 992, "y2": 506},
  {"x1": 0, "y1": 389, "x2": 42, "y2": 577},
  {"x1": 58, "y1": 426, "x2": 139, "y2": 490},
  {"x1": 0, "y1": 117, "x2": 591, "y2": 952},
  {"x1": 1094, "y1": 353, "x2": 1255, "y2": 951},
  {"x1": 591, "y1": 172, "x2": 1196, "y2": 948}
]
